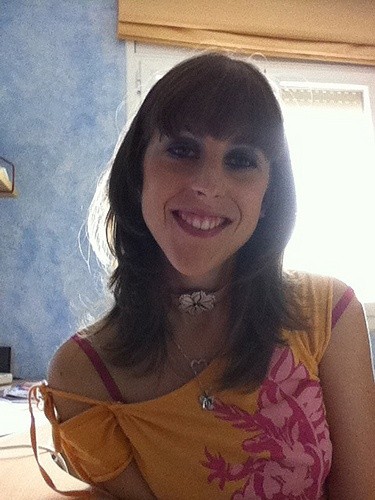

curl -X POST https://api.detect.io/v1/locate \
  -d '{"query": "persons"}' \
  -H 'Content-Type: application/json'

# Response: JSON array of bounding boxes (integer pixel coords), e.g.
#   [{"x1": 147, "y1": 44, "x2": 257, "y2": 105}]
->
[{"x1": 26, "y1": 47, "x2": 374, "y2": 500}]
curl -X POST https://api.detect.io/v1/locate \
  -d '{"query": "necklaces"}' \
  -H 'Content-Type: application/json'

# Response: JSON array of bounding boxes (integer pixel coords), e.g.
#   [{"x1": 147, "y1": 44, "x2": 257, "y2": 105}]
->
[
  {"x1": 168, "y1": 326, "x2": 238, "y2": 413},
  {"x1": 165, "y1": 280, "x2": 232, "y2": 317}
]
